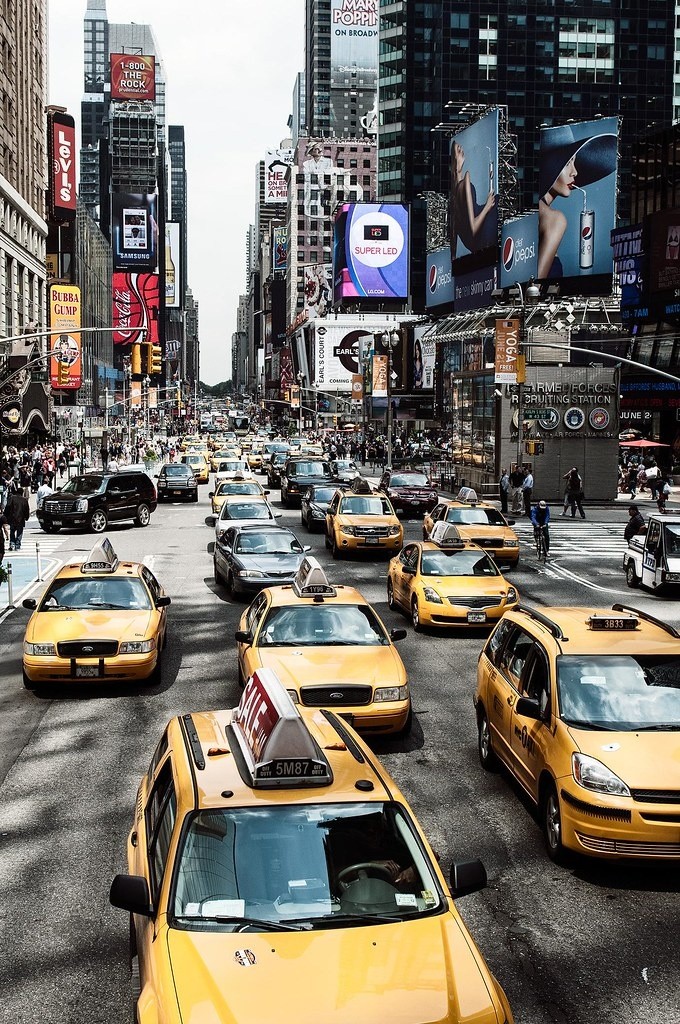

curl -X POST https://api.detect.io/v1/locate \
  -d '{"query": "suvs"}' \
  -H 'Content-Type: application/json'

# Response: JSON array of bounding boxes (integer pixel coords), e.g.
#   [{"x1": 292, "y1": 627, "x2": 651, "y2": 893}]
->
[
  {"x1": 36, "y1": 470, "x2": 156, "y2": 534},
  {"x1": 473, "y1": 604, "x2": 680, "y2": 860},
  {"x1": 323, "y1": 479, "x2": 403, "y2": 558}
]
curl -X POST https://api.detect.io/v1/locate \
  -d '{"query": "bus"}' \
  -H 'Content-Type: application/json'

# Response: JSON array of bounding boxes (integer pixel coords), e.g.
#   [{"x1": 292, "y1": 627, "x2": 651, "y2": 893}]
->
[
  {"x1": 227, "y1": 412, "x2": 249, "y2": 436},
  {"x1": 200, "y1": 404, "x2": 230, "y2": 431}
]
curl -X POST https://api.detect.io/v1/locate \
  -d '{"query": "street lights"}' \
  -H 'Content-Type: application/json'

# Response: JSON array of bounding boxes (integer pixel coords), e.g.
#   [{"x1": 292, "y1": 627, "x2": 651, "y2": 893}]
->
[
  {"x1": 381, "y1": 328, "x2": 400, "y2": 464},
  {"x1": 142, "y1": 377, "x2": 152, "y2": 440},
  {"x1": 512, "y1": 280, "x2": 539, "y2": 464}
]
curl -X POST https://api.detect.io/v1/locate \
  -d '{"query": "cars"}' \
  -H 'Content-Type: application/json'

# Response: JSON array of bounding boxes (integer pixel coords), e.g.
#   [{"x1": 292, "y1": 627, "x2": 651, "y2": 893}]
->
[
  {"x1": 422, "y1": 486, "x2": 519, "y2": 570},
  {"x1": 155, "y1": 431, "x2": 439, "y2": 533},
  {"x1": 215, "y1": 524, "x2": 311, "y2": 596},
  {"x1": 21, "y1": 538, "x2": 171, "y2": 687},
  {"x1": 387, "y1": 521, "x2": 522, "y2": 631},
  {"x1": 236, "y1": 554, "x2": 410, "y2": 736},
  {"x1": 252, "y1": 421, "x2": 279, "y2": 436},
  {"x1": 108, "y1": 668, "x2": 512, "y2": 1024}
]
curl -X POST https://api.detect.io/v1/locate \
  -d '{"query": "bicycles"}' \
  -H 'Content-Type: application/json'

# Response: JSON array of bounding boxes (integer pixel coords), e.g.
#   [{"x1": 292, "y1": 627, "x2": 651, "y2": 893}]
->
[{"x1": 534, "y1": 525, "x2": 551, "y2": 563}]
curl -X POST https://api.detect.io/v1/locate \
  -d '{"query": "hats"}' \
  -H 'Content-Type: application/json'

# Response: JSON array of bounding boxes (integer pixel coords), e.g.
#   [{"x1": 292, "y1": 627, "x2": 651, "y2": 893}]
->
[{"x1": 539, "y1": 501, "x2": 546, "y2": 509}]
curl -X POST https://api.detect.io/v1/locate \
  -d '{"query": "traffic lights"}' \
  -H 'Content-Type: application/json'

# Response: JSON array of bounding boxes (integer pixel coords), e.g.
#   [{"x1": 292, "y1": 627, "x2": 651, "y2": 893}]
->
[
  {"x1": 146, "y1": 344, "x2": 162, "y2": 375},
  {"x1": 285, "y1": 391, "x2": 290, "y2": 402},
  {"x1": 529, "y1": 440, "x2": 544, "y2": 455},
  {"x1": 332, "y1": 415, "x2": 337, "y2": 424},
  {"x1": 226, "y1": 400, "x2": 230, "y2": 406},
  {"x1": 178, "y1": 402, "x2": 181, "y2": 408},
  {"x1": 58, "y1": 361, "x2": 70, "y2": 384}
]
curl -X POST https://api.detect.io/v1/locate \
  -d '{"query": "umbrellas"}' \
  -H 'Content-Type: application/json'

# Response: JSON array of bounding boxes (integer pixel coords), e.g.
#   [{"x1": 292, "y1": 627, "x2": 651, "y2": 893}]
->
[{"x1": 618, "y1": 439, "x2": 669, "y2": 462}]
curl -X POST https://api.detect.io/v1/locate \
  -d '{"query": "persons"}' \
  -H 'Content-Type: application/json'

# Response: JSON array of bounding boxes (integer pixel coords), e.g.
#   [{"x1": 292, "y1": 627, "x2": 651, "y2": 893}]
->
[
  {"x1": 411, "y1": 338, "x2": 425, "y2": 388},
  {"x1": 541, "y1": 123, "x2": 617, "y2": 280},
  {"x1": 304, "y1": 141, "x2": 332, "y2": 170},
  {"x1": 498, "y1": 452, "x2": 670, "y2": 557},
  {"x1": 451, "y1": 141, "x2": 498, "y2": 260},
  {"x1": 0, "y1": 416, "x2": 199, "y2": 566},
  {"x1": 331, "y1": 825, "x2": 421, "y2": 895},
  {"x1": 309, "y1": 422, "x2": 448, "y2": 472}
]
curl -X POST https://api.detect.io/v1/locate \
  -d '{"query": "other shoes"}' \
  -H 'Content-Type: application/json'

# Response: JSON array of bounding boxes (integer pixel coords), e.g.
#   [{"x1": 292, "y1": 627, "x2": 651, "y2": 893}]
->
[
  {"x1": 546, "y1": 552, "x2": 550, "y2": 557},
  {"x1": 559, "y1": 512, "x2": 565, "y2": 516},
  {"x1": 569, "y1": 514, "x2": 575, "y2": 518}
]
